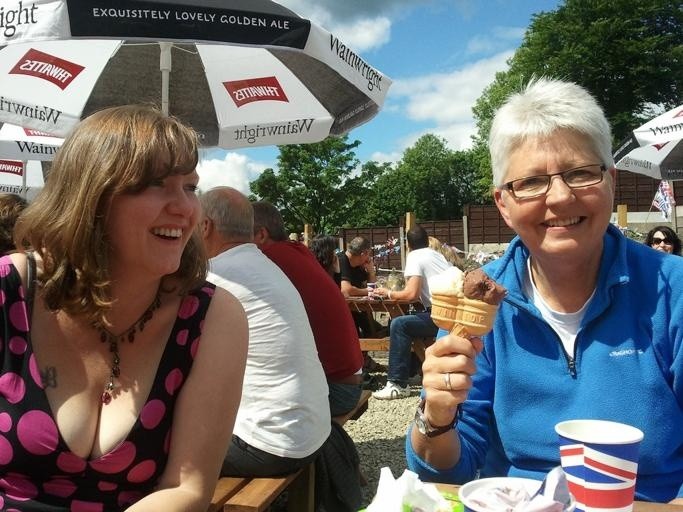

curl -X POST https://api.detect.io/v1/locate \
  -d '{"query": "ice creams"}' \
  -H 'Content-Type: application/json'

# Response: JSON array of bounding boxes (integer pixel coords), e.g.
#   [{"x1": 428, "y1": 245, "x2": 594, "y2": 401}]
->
[{"x1": 427, "y1": 266, "x2": 506, "y2": 341}]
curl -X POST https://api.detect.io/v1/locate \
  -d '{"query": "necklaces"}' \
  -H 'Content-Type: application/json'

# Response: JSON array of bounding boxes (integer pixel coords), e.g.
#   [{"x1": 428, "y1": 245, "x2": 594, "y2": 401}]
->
[
  {"x1": 91, "y1": 291, "x2": 161, "y2": 404},
  {"x1": 214, "y1": 242, "x2": 248, "y2": 256}
]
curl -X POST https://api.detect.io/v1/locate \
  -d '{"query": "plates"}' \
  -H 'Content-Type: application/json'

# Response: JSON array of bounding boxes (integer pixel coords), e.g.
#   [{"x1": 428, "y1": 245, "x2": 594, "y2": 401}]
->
[{"x1": 457, "y1": 476, "x2": 576, "y2": 512}]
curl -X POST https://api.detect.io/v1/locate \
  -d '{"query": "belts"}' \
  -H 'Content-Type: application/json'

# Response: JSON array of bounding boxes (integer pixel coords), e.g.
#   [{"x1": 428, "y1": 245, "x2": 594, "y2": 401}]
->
[{"x1": 333, "y1": 374, "x2": 364, "y2": 385}]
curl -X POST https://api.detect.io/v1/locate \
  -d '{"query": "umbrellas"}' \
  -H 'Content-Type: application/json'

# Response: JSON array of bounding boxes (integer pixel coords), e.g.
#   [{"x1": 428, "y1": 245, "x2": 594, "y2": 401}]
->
[
  {"x1": 612, "y1": 105, "x2": 683, "y2": 181},
  {"x1": 0, "y1": 159, "x2": 45, "y2": 204},
  {"x1": 0, "y1": 0, "x2": 393, "y2": 150},
  {"x1": 0, "y1": 123, "x2": 65, "y2": 199}
]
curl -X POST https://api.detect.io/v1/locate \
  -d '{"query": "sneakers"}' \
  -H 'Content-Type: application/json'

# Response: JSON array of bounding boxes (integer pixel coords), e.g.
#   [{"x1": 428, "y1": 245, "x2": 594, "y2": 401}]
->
[
  {"x1": 373, "y1": 380, "x2": 411, "y2": 400},
  {"x1": 408, "y1": 374, "x2": 422, "y2": 385}
]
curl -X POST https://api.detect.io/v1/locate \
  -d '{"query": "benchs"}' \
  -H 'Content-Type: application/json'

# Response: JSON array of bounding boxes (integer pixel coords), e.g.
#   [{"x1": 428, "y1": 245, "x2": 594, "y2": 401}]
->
[{"x1": 209, "y1": 389, "x2": 371, "y2": 511}]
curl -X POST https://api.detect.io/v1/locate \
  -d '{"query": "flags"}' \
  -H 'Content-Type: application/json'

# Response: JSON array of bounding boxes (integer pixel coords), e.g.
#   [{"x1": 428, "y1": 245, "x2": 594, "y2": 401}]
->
[{"x1": 652, "y1": 180, "x2": 676, "y2": 222}]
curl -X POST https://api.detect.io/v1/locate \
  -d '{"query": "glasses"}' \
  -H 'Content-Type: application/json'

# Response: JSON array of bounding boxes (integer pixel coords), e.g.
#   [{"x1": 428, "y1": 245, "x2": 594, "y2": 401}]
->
[
  {"x1": 650, "y1": 236, "x2": 673, "y2": 246},
  {"x1": 498, "y1": 161, "x2": 606, "y2": 201}
]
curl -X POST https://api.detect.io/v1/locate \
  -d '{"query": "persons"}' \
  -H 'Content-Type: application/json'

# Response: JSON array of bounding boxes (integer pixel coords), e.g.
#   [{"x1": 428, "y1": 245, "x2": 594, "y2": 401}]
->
[
  {"x1": 0, "y1": 104, "x2": 248, "y2": 512},
  {"x1": 252, "y1": 201, "x2": 364, "y2": 415},
  {"x1": 0, "y1": 192, "x2": 30, "y2": 258},
  {"x1": 646, "y1": 225, "x2": 681, "y2": 256},
  {"x1": 309, "y1": 224, "x2": 464, "y2": 400},
  {"x1": 198, "y1": 185, "x2": 332, "y2": 478},
  {"x1": 405, "y1": 71, "x2": 683, "y2": 512}
]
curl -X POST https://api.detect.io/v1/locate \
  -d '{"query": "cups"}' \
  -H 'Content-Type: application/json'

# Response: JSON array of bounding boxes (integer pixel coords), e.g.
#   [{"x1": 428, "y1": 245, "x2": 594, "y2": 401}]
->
[
  {"x1": 366, "y1": 282, "x2": 376, "y2": 300},
  {"x1": 553, "y1": 417, "x2": 645, "y2": 512}
]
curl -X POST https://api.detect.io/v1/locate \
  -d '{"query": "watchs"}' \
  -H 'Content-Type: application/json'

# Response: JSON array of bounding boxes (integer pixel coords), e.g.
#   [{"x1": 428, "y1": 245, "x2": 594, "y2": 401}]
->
[{"x1": 414, "y1": 400, "x2": 463, "y2": 438}]
